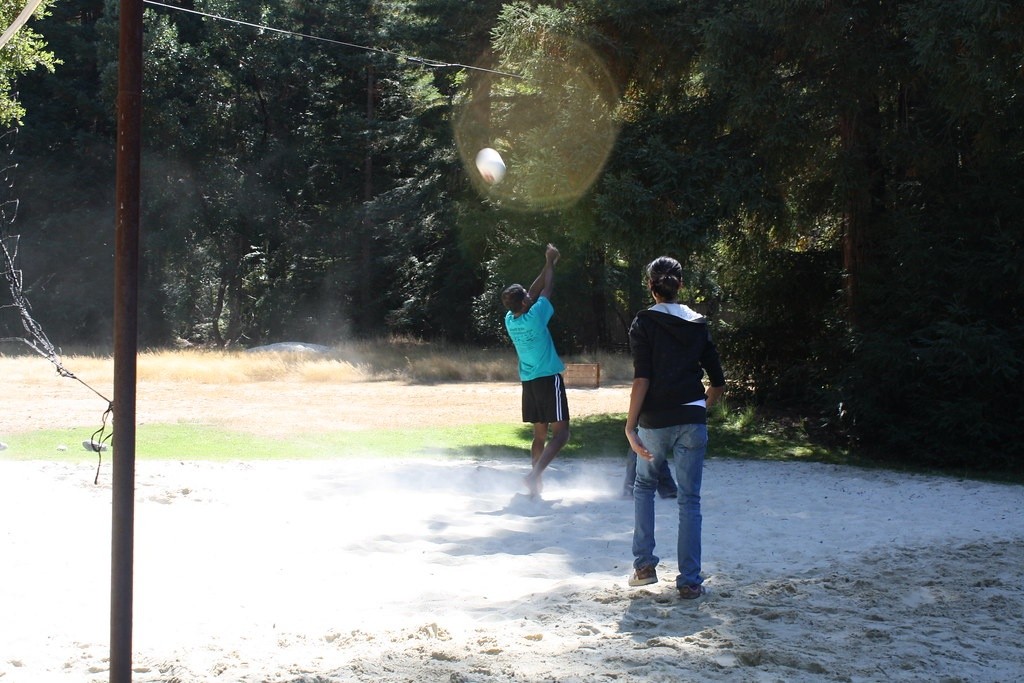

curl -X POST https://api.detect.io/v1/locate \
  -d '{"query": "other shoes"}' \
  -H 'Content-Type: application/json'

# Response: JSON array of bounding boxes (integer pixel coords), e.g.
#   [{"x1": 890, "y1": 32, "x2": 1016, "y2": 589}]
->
[
  {"x1": 681, "y1": 583, "x2": 711, "y2": 601},
  {"x1": 627, "y1": 564, "x2": 658, "y2": 588}
]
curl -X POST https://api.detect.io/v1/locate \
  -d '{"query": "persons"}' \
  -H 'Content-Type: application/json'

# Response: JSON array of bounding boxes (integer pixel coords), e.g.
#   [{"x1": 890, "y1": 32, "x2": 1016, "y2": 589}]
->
[
  {"x1": 620, "y1": 255, "x2": 726, "y2": 599},
  {"x1": 502, "y1": 243, "x2": 570, "y2": 494}
]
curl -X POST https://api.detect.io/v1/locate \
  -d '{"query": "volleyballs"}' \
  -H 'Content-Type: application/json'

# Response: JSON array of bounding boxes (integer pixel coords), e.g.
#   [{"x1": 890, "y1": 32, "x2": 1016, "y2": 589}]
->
[{"x1": 475, "y1": 146, "x2": 509, "y2": 186}]
